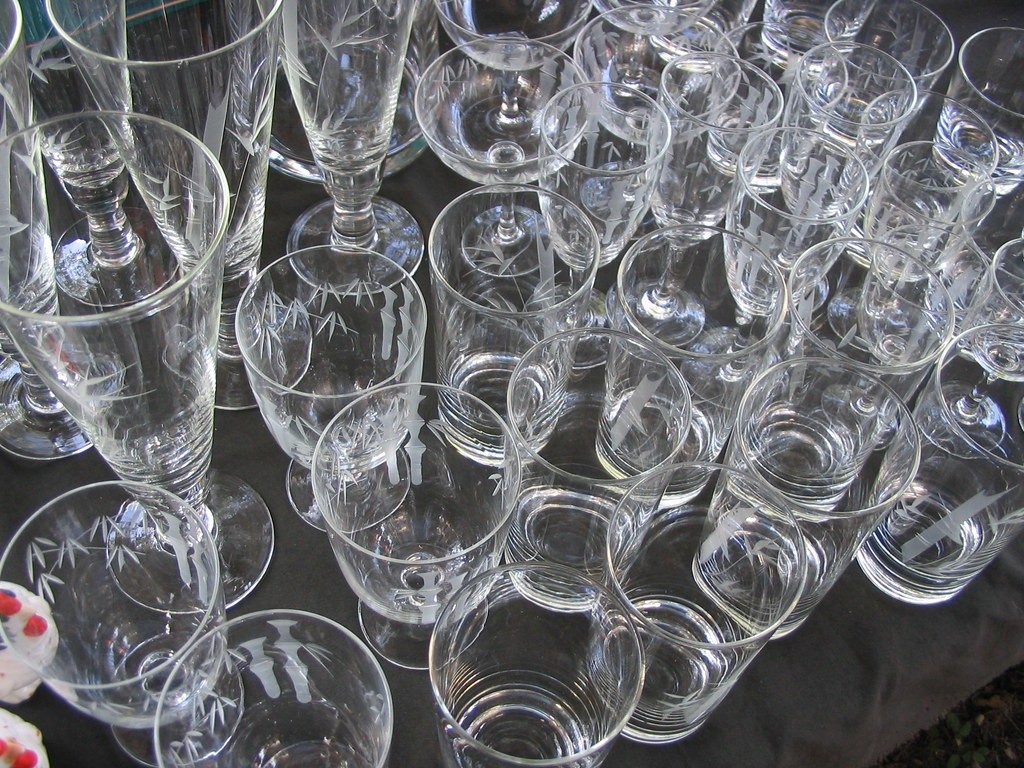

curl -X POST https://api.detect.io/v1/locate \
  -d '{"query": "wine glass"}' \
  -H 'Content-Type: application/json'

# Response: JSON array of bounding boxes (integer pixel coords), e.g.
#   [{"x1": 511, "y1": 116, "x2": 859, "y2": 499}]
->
[{"x1": 0, "y1": 0, "x2": 1024, "y2": 768}]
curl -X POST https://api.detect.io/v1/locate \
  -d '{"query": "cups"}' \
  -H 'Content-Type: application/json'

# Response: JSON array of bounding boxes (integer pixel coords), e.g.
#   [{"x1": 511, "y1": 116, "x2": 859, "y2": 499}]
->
[
  {"x1": 427, "y1": 183, "x2": 595, "y2": 464},
  {"x1": 731, "y1": 237, "x2": 956, "y2": 519},
  {"x1": 691, "y1": 357, "x2": 921, "y2": 638},
  {"x1": 590, "y1": 462, "x2": 808, "y2": 745},
  {"x1": 933, "y1": 27, "x2": 1024, "y2": 198},
  {"x1": 648, "y1": 0, "x2": 753, "y2": 74},
  {"x1": 502, "y1": 330, "x2": 691, "y2": 612},
  {"x1": 597, "y1": 224, "x2": 788, "y2": 501},
  {"x1": 856, "y1": 324, "x2": 1023, "y2": 607},
  {"x1": 762, "y1": 0, "x2": 876, "y2": 75},
  {"x1": 429, "y1": 562, "x2": 646, "y2": 767}
]
